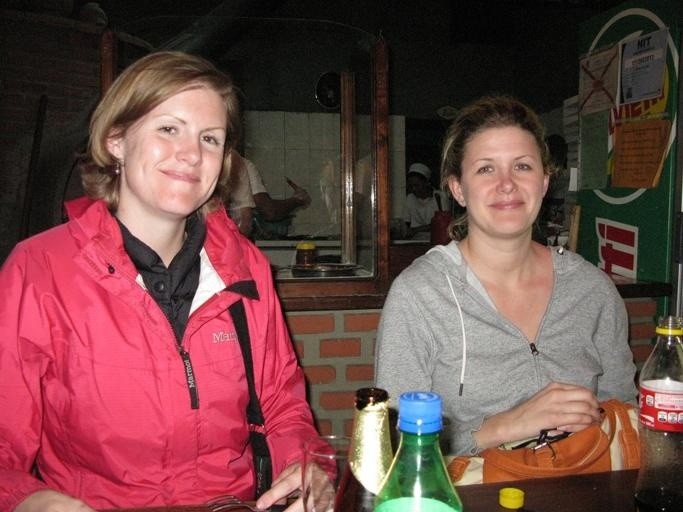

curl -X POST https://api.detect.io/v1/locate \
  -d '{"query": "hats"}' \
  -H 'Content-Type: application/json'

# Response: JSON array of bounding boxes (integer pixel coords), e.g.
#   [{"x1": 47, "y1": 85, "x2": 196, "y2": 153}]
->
[{"x1": 409, "y1": 163, "x2": 432, "y2": 181}]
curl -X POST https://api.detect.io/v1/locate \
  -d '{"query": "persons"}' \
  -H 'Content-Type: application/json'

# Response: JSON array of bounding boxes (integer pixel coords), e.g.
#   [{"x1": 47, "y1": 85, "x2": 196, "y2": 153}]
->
[
  {"x1": 240, "y1": 154, "x2": 311, "y2": 223},
  {"x1": 0, "y1": 48, "x2": 338, "y2": 511},
  {"x1": 228, "y1": 158, "x2": 257, "y2": 239},
  {"x1": 369, "y1": 96, "x2": 639, "y2": 456},
  {"x1": 399, "y1": 161, "x2": 449, "y2": 232}
]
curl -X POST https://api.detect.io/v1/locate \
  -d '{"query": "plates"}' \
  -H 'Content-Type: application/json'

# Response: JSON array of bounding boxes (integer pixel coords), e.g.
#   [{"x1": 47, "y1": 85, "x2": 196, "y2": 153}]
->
[
  {"x1": 269, "y1": 263, "x2": 285, "y2": 279},
  {"x1": 287, "y1": 264, "x2": 366, "y2": 277}
]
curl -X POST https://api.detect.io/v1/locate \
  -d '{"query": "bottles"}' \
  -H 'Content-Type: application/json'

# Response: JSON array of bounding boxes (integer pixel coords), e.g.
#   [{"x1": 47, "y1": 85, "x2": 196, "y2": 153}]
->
[
  {"x1": 330, "y1": 386, "x2": 396, "y2": 512},
  {"x1": 631, "y1": 313, "x2": 683, "y2": 512},
  {"x1": 431, "y1": 210, "x2": 454, "y2": 246},
  {"x1": 368, "y1": 388, "x2": 466, "y2": 512},
  {"x1": 294, "y1": 243, "x2": 318, "y2": 264}
]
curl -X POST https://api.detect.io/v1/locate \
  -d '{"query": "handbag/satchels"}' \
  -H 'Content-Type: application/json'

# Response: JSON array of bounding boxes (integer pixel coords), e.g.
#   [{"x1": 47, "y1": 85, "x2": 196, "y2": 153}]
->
[{"x1": 443, "y1": 400, "x2": 641, "y2": 486}]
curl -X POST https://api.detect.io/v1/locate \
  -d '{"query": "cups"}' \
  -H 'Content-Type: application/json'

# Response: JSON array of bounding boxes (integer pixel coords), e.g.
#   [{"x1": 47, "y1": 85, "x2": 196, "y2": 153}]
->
[{"x1": 300, "y1": 432, "x2": 362, "y2": 512}]
